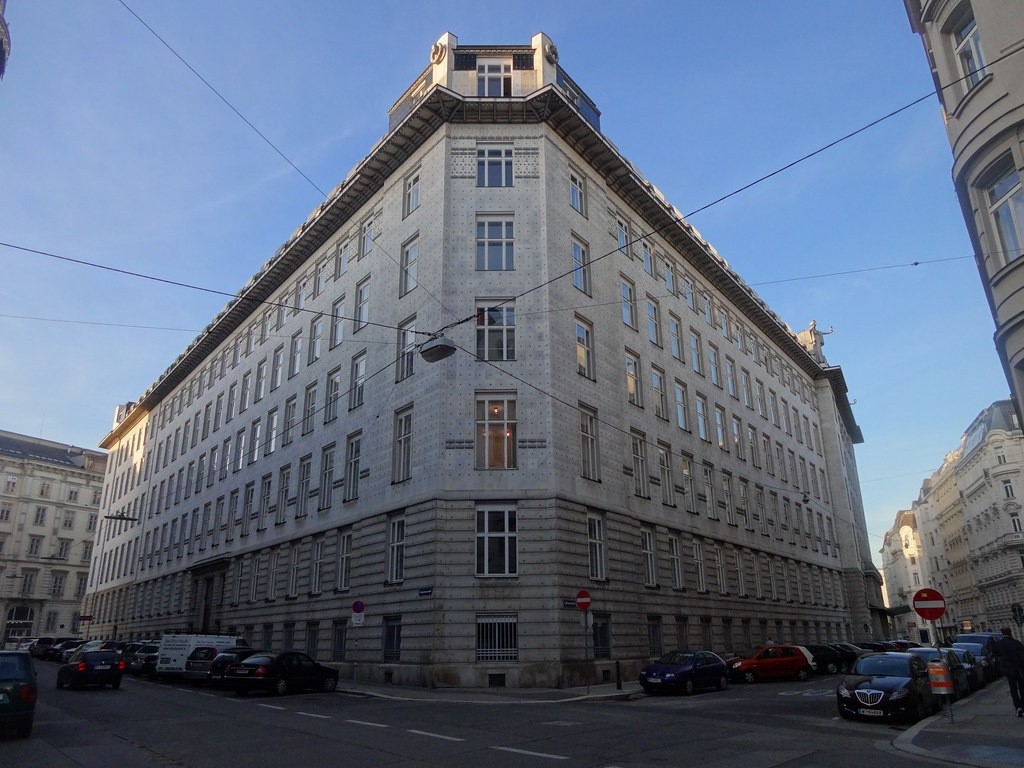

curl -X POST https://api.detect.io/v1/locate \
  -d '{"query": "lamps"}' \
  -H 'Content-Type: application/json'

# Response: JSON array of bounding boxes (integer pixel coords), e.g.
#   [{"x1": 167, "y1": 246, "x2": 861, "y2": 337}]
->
[
  {"x1": 802, "y1": 494, "x2": 810, "y2": 504},
  {"x1": 104, "y1": 513, "x2": 138, "y2": 522},
  {"x1": 419, "y1": 332, "x2": 458, "y2": 363},
  {"x1": 41, "y1": 555, "x2": 65, "y2": 560},
  {"x1": 6, "y1": 574, "x2": 24, "y2": 578}
]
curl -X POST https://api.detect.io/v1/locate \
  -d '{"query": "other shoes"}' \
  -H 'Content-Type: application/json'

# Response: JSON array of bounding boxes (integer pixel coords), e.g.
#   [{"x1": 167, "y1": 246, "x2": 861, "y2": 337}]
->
[{"x1": 1016, "y1": 707, "x2": 1022, "y2": 717}]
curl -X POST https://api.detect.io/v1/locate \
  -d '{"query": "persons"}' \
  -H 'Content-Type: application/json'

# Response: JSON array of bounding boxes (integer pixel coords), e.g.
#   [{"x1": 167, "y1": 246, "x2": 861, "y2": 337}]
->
[{"x1": 993, "y1": 626, "x2": 1024, "y2": 718}]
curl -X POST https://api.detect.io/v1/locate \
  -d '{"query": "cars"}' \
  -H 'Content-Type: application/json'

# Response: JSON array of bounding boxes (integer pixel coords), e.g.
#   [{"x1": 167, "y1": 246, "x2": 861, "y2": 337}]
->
[
  {"x1": 638, "y1": 650, "x2": 730, "y2": 696},
  {"x1": 0, "y1": 650, "x2": 38, "y2": 737},
  {"x1": 4, "y1": 634, "x2": 339, "y2": 698},
  {"x1": 836, "y1": 632, "x2": 1004, "y2": 724},
  {"x1": 726, "y1": 645, "x2": 817, "y2": 684},
  {"x1": 803, "y1": 640, "x2": 925, "y2": 674}
]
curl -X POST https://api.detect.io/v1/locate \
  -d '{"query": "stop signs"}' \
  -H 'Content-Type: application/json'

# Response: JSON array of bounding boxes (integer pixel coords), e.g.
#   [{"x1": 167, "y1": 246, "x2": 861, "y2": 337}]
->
[
  {"x1": 577, "y1": 590, "x2": 591, "y2": 611},
  {"x1": 912, "y1": 588, "x2": 946, "y2": 621}
]
